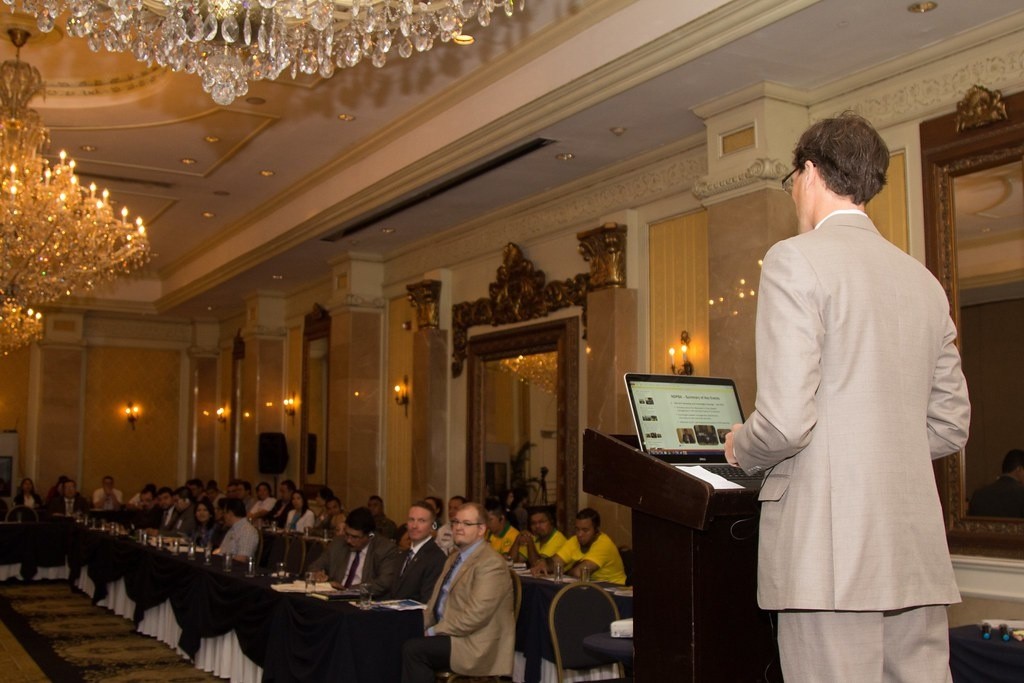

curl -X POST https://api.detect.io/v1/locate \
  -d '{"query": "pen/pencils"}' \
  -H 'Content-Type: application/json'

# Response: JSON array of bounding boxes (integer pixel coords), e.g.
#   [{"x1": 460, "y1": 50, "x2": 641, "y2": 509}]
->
[{"x1": 322, "y1": 568, "x2": 326, "y2": 577}]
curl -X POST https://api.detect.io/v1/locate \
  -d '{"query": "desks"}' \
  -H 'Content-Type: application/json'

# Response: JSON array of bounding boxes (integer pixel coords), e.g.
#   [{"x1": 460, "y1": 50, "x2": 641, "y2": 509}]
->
[{"x1": 0, "y1": 511, "x2": 633, "y2": 683}]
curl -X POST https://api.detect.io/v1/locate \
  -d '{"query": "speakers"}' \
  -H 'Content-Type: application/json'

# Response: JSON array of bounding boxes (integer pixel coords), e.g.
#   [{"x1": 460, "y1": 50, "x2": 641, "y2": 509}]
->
[
  {"x1": 306, "y1": 433, "x2": 318, "y2": 475},
  {"x1": 258, "y1": 433, "x2": 289, "y2": 475}
]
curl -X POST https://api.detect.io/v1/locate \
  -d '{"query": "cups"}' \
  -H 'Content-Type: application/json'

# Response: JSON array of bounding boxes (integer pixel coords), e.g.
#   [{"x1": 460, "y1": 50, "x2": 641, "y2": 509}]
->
[
  {"x1": 277, "y1": 562, "x2": 288, "y2": 582},
  {"x1": 91, "y1": 517, "x2": 97, "y2": 528},
  {"x1": 554, "y1": 562, "x2": 588, "y2": 585},
  {"x1": 245, "y1": 556, "x2": 254, "y2": 577},
  {"x1": 248, "y1": 516, "x2": 262, "y2": 530},
  {"x1": 204, "y1": 547, "x2": 211, "y2": 565},
  {"x1": 137, "y1": 528, "x2": 148, "y2": 545},
  {"x1": 173, "y1": 538, "x2": 180, "y2": 557},
  {"x1": 157, "y1": 534, "x2": 163, "y2": 550},
  {"x1": 285, "y1": 520, "x2": 291, "y2": 534},
  {"x1": 271, "y1": 521, "x2": 277, "y2": 533},
  {"x1": 304, "y1": 526, "x2": 310, "y2": 536},
  {"x1": 360, "y1": 582, "x2": 372, "y2": 609},
  {"x1": 100, "y1": 518, "x2": 106, "y2": 531},
  {"x1": 323, "y1": 528, "x2": 328, "y2": 538},
  {"x1": 188, "y1": 544, "x2": 197, "y2": 560},
  {"x1": 76, "y1": 511, "x2": 89, "y2": 526},
  {"x1": 223, "y1": 551, "x2": 232, "y2": 572},
  {"x1": 110, "y1": 523, "x2": 115, "y2": 534},
  {"x1": 305, "y1": 572, "x2": 315, "y2": 596}
]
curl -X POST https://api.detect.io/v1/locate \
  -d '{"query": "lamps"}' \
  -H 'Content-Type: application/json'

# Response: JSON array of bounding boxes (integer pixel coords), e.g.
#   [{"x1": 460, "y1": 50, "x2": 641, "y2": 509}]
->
[
  {"x1": 217, "y1": 407, "x2": 227, "y2": 431},
  {"x1": 0, "y1": 0, "x2": 526, "y2": 106},
  {"x1": 0, "y1": 13, "x2": 160, "y2": 358},
  {"x1": 124, "y1": 402, "x2": 139, "y2": 431},
  {"x1": 282, "y1": 397, "x2": 295, "y2": 423},
  {"x1": 668, "y1": 330, "x2": 694, "y2": 376},
  {"x1": 394, "y1": 375, "x2": 409, "y2": 417}
]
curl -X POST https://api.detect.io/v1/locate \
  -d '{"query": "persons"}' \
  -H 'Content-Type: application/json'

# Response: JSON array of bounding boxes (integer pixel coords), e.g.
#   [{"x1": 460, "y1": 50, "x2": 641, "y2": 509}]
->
[
  {"x1": 530, "y1": 506, "x2": 627, "y2": 585},
  {"x1": 968, "y1": 448, "x2": 1024, "y2": 518},
  {"x1": 374, "y1": 502, "x2": 447, "y2": 605},
  {"x1": 397, "y1": 501, "x2": 515, "y2": 683},
  {"x1": 305, "y1": 505, "x2": 402, "y2": 596},
  {"x1": 484, "y1": 483, "x2": 530, "y2": 530},
  {"x1": 125, "y1": 482, "x2": 162, "y2": 510},
  {"x1": 509, "y1": 507, "x2": 575, "y2": 575},
  {"x1": 183, "y1": 477, "x2": 349, "y2": 538},
  {"x1": 484, "y1": 501, "x2": 521, "y2": 559},
  {"x1": 153, "y1": 489, "x2": 260, "y2": 564},
  {"x1": 394, "y1": 497, "x2": 445, "y2": 544},
  {"x1": 11, "y1": 474, "x2": 90, "y2": 518},
  {"x1": 724, "y1": 105, "x2": 971, "y2": 683},
  {"x1": 90, "y1": 477, "x2": 123, "y2": 511},
  {"x1": 433, "y1": 496, "x2": 466, "y2": 556},
  {"x1": 366, "y1": 495, "x2": 398, "y2": 537}
]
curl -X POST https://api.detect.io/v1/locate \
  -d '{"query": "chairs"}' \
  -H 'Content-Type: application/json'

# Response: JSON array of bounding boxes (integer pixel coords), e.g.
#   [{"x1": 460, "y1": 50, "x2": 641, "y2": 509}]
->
[
  {"x1": 0, "y1": 504, "x2": 306, "y2": 683},
  {"x1": 436, "y1": 568, "x2": 522, "y2": 683},
  {"x1": 547, "y1": 581, "x2": 632, "y2": 683}
]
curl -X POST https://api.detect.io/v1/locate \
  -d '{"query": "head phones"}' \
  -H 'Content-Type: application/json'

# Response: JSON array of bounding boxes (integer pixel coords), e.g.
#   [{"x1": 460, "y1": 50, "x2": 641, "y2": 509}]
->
[
  {"x1": 369, "y1": 532, "x2": 375, "y2": 539},
  {"x1": 432, "y1": 521, "x2": 437, "y2": 530}
]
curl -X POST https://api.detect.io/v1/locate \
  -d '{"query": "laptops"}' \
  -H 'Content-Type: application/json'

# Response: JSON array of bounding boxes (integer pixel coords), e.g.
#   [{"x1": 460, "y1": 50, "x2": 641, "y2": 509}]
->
[{"x1": 624, "y1": 372, "x2": 767, "y2": 493}]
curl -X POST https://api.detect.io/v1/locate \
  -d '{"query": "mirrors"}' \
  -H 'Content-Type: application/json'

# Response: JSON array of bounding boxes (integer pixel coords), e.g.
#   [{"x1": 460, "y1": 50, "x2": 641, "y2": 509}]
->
[{"x1": 918, "y1": 85, "x2": 1024, "y2": 560}]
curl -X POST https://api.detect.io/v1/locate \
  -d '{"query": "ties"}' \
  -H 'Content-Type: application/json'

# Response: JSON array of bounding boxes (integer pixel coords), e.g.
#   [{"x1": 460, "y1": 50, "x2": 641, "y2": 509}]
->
[
  {"x1": 433, "y1": 554, "x2": 463, "y2": 624},
  {"x1": 399, "y1": 549, "x2": 415, "y2": 579},
  {"x1": 67, "y1": 500, "x2": 72, "y2": 517},
  {"x1": 344, "y1": 550, "x2": 363, "y2": 588},
  {"x1": 162, "y1": 511, "x2": 169, "y2": 525}
]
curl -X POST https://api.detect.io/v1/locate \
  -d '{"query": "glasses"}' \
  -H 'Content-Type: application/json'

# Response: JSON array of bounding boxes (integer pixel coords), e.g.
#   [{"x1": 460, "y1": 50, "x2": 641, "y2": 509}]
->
[
  {"x1": 448, "y1": 519, "x2": 484, "y2": 528},
  {"x1": 780, "y1": 163, "x2": 816, "y2": 197},
  {"x1": 343, "y1": 531, "x2": 365, "y2": 541}
]
